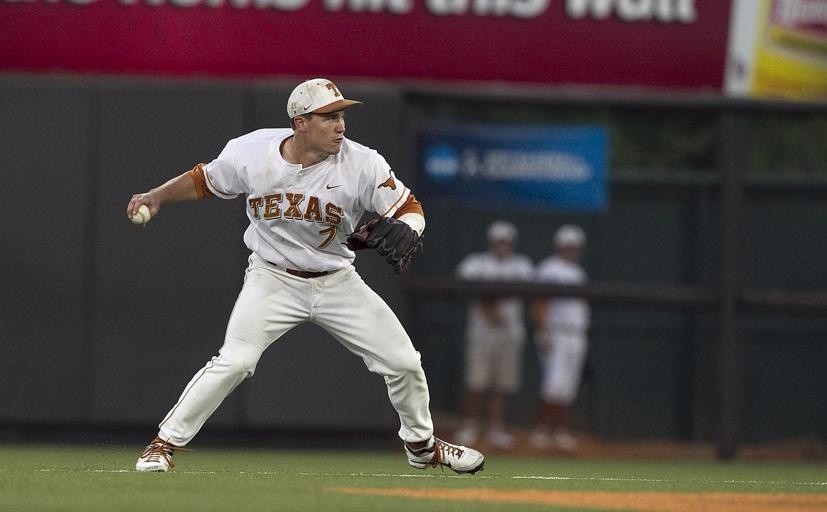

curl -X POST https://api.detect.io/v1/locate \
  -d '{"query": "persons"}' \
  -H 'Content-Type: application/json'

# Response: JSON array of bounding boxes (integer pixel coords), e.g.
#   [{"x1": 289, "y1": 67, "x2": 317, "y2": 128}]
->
[
  {"x1": 454, "y1": 220, "x2": 534, "y2": 449},
  {"x1": 528, "y1": 224, "x2": 593, "y2": 451},
  {"x1": 126, "y1": 78, "x2": 486, "y2": 474}
]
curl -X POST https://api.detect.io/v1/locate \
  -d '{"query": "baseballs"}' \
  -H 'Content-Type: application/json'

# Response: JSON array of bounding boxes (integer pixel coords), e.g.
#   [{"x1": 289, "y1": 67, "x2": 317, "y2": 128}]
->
[{"x1": 127, "y1": 204, "x2": 152, "y2": 225}]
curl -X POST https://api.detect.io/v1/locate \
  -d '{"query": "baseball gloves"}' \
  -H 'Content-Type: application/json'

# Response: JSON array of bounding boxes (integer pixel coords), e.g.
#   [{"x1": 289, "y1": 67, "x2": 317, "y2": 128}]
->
[{"x1": 343, "y1": 216, "x2": 424, "y2": 274}]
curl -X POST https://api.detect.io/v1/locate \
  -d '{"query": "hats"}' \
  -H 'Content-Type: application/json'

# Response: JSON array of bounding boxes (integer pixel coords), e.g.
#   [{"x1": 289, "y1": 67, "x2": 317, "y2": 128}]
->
[{"x1": 286, "y1": 78, "x2": 364, "y2": 121}]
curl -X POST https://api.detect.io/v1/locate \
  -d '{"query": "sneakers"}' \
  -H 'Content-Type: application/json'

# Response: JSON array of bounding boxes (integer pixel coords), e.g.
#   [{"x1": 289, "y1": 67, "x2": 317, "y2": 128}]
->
[
  {"x1": 403, "y1": 434, "x2": 485, "y2": 474},
  {"x1": 135, "y1": 439, "x2": 193, "y2": 472}
]
